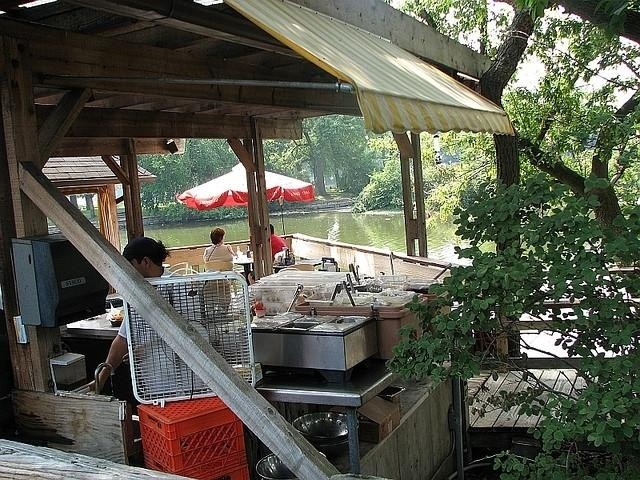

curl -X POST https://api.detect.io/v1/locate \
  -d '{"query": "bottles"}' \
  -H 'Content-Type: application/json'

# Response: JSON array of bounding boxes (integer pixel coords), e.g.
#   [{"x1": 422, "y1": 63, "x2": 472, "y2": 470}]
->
[
  {"x1": 247, "y1": 246, "x2": 251, "y2": 258},
  {"x1": 236, "y1": 245, "x2": 241, "y2": 258}
]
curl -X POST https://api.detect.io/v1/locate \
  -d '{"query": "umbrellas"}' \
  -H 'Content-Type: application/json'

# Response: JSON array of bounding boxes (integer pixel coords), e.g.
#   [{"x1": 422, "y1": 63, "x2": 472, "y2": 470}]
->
[{"x1": 175, "y1": 162, "x2": 315, "y2": 210}]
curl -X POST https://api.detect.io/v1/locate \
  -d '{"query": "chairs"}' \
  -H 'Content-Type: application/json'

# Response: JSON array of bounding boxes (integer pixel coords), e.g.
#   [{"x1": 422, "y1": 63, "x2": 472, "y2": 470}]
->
[{"x1": 203, "y1": 260, "x2": 233, "y2": 273}]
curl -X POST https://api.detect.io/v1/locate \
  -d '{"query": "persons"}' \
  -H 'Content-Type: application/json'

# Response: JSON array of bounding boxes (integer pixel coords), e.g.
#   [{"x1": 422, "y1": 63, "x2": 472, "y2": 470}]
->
[
  {"x1": 98, "y1": 237, "x2": 199, "y2": 390},
  {"x1": 203, "y1": 228, "x2": 235, "y2": 263},
  {"x1": 247, "y1": 223, "x2": 287, "y2": 284}
]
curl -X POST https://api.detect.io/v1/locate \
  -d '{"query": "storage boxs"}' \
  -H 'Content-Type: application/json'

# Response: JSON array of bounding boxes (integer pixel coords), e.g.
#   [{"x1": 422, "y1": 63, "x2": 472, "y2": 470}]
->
[{"x1": 135, "y1": 396, "x2": 251, "y2": 479}]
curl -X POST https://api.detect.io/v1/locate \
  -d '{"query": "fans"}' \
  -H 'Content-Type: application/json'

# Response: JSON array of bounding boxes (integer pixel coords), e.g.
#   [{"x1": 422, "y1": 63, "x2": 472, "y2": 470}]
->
[{"x1": 118, "y1": 271, "x2": 255, "y2": 405}]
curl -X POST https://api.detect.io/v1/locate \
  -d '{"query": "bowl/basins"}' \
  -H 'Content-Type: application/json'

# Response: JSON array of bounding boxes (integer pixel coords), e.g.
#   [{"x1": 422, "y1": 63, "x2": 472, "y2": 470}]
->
[
  {"x1": 255, "y1": 452, "x2": 327, "y2": 480},
  {"x1": 291, "y1": 410, "x2": 359, "y2": 458}
]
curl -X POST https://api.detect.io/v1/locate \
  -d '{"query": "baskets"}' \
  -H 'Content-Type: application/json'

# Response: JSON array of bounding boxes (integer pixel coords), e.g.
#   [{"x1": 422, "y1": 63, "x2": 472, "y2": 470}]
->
[{"x1": 137, "y1": 397, "x2": 251, "y2": 480}]
